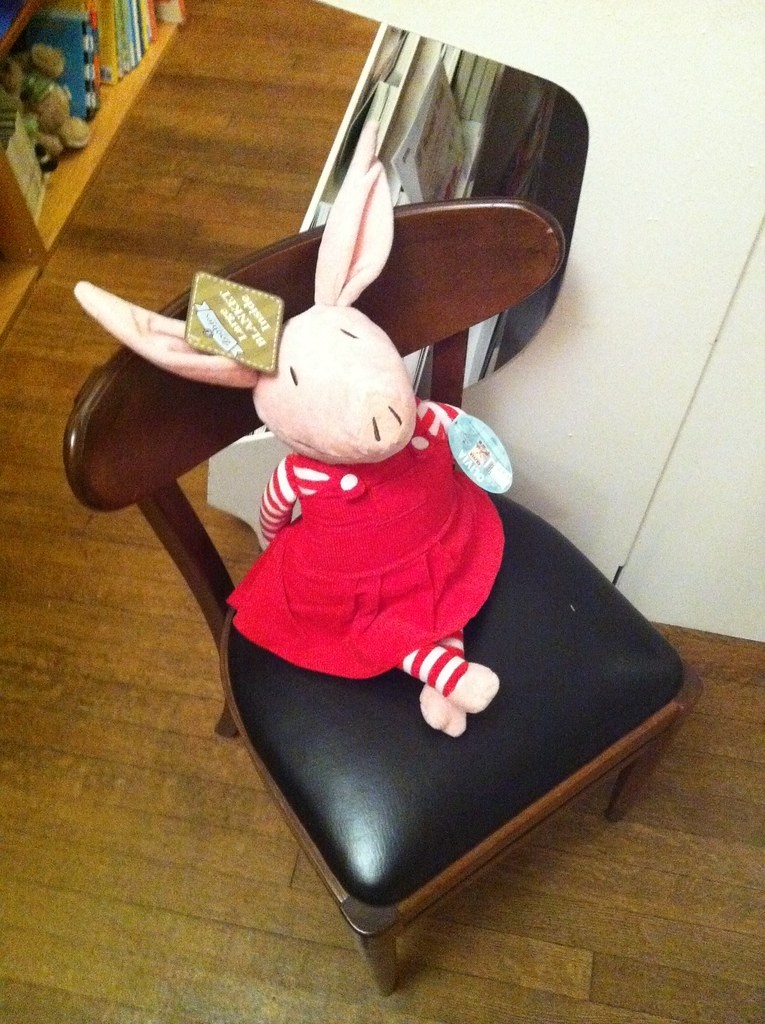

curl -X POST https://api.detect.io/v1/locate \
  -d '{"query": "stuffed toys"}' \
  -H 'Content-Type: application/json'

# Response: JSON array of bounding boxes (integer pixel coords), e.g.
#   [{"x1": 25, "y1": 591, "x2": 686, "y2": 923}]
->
[
  {"x1": 0, "y1": 43, "x2": 90, "y2": 171},
  {"x1": 334, "y1": 25, "x2": 502, "y2": 204},
  {"x1": 72, "y1": 160, "x2": 513, "y2": 739}
]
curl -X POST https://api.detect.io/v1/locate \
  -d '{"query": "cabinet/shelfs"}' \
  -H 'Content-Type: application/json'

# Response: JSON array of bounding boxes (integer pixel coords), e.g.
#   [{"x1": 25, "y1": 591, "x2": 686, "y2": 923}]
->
[{"x1": 1, "y1": 0, "x2": 186, "y2": 339}]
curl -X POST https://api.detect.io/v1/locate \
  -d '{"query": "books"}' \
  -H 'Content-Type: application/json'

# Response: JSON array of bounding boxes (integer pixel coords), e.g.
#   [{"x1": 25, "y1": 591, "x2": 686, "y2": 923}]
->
[{"x1": 24, "y1": 0, "x2": 187, "y2": 123}]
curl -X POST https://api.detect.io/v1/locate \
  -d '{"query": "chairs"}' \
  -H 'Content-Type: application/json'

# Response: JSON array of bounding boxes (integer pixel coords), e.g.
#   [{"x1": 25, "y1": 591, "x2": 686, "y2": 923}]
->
[{"x1": 62, "y1": 199, "x2": 704, "y2": 999}]
